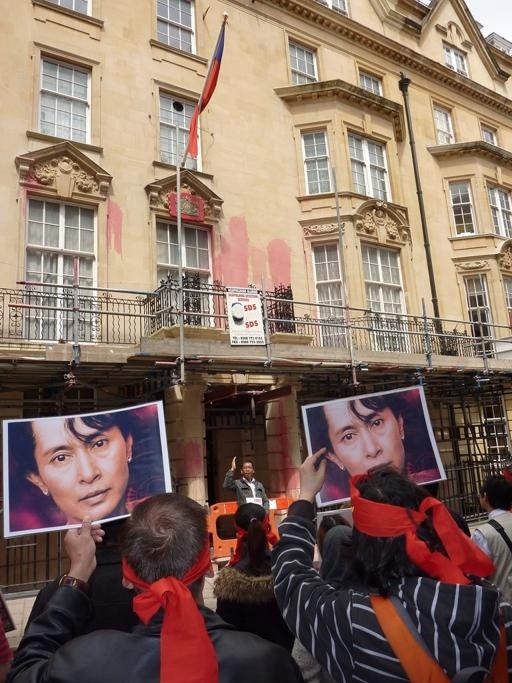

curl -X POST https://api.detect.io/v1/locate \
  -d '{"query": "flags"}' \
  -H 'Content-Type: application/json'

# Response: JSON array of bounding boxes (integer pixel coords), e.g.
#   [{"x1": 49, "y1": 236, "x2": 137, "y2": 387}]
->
[{"x1": 188, "y1": 21, "x2": 225, "y2": 159}]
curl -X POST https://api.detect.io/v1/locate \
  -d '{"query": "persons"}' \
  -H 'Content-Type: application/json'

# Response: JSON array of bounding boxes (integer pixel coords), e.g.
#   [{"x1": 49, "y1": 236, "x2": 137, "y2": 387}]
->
[
  {"x1": 0, "y1": 449, "x2": 511, "y2": 683},
  {"x1": 13, "y1": 411, "x2": 139, "y2": 526},
  {"x1": 315, "y1": 392, "x2": 441, "y2": 502}
]
